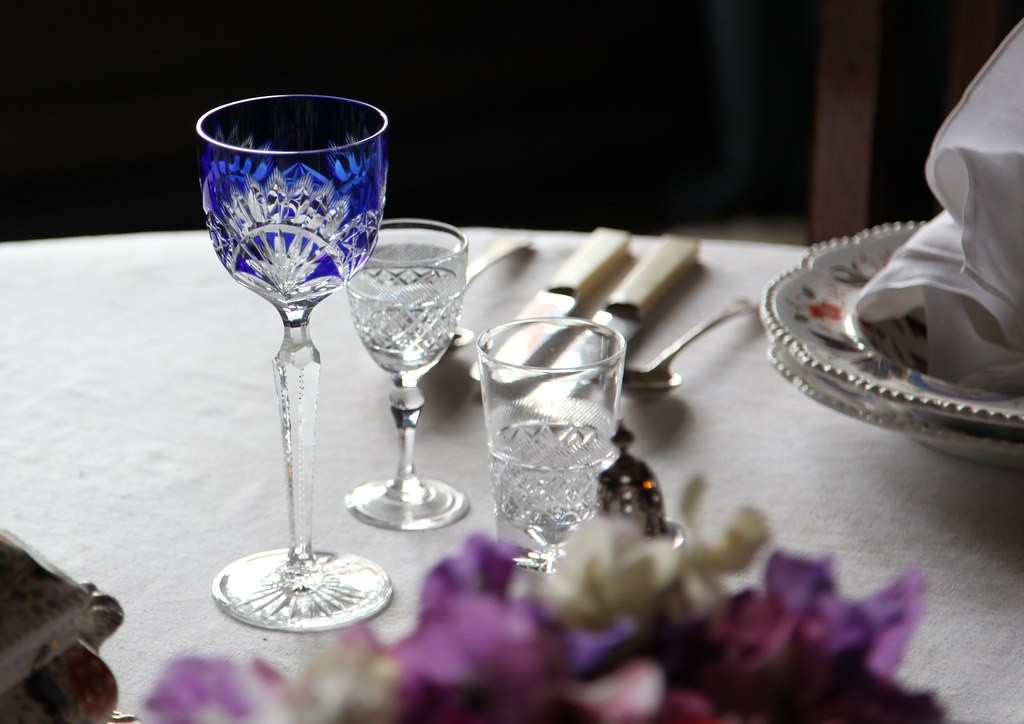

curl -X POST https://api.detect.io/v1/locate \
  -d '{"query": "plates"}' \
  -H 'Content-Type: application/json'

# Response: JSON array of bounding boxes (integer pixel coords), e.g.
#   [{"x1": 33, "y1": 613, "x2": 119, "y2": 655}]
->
[{"x1": 762, "y1": 219, "x2": 1023, "y2": 469}]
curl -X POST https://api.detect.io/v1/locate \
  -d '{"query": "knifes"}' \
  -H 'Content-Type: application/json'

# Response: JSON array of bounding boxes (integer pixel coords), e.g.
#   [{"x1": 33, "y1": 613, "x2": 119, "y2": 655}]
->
[
  {"x1": 528, "y1": 234, "x2": 706, "y2": 407},
  {"x1": 476, "y1": 227, "x2": 633, "y2": 383}
]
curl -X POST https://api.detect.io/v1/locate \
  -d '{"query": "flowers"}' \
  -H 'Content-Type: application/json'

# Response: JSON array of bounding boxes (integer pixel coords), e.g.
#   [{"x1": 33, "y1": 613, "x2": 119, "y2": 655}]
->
[{"x1": 140, "y1": 470, "x2": 949, "y2": 723}]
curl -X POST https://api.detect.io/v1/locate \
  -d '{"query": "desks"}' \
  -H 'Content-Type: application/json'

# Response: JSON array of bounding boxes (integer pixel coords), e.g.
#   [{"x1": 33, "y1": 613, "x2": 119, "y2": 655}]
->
[{"x1": 1, "y1": 226, "x2": 1023, "y2": 724}]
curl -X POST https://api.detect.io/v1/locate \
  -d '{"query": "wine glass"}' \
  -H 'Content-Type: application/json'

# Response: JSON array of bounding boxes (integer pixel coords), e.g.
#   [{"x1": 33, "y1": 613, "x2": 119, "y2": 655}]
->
[
  {"x1": 345, "y1": 218, "x2": 470, "y2": 529},
  {"x1": 196, "y1": 93, "x2": 393, "y2": 629}
]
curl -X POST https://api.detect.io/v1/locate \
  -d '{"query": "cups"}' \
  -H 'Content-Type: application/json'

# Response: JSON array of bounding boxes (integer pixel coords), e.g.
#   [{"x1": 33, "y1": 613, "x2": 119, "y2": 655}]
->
[{"x1": 476, "y1": 317, "x2": 628, "y2": 576}]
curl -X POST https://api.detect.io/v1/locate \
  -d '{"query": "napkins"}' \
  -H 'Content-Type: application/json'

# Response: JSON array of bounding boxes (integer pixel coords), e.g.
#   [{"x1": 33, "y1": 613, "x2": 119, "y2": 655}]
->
[{"x1": 855, "y1": 21, "x2": 1024, "y2": 389}]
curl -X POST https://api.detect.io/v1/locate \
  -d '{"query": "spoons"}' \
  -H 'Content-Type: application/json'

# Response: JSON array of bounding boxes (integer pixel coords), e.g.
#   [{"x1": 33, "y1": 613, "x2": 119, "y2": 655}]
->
[
  {"x1": 398, "y1": 237, "x2": 524, "y2": 356},
  {"x1": 625, "y1": 295, "x2": 755, "y2": 389}
]
